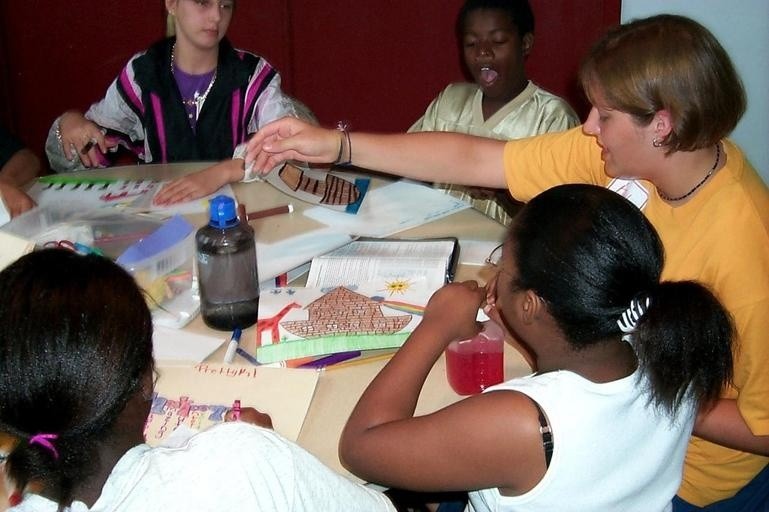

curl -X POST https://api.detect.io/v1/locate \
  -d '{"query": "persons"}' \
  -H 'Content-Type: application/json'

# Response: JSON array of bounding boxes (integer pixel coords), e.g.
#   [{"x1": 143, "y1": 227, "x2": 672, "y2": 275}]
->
[
  {"x1": 333, "y1": 178, "x2": 744, "y2": 511},
  {"x1": 239, "y1": 10, "x2": 768, "y2": 511},
  {"x1": 42, "y1": 0, "x2": 301, "y2": 213},
  {"x1": 1, "y1": 138, "x2": 46, "y2": 226},
  {"x1": 1, "y1": 244, "x2": 406, "y2": 511},
  {"x1": 393, "y1": 0, "x2": 584, "y2": 238}
]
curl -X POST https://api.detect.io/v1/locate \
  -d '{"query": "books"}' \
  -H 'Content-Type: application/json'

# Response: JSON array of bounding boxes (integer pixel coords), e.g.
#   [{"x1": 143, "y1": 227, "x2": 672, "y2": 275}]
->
[{"x1": 306, "y1": 233, "x2": 462, "y2": 317}]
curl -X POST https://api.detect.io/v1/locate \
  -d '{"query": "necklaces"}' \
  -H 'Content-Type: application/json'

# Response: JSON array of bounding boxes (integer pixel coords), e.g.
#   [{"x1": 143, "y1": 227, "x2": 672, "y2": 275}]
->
[
  {"x1": 170, "y1": 42, "x2": 219, "y2": 106},
  {"x1": 654, "y1": 140, "x2": 721, "y2": 202}
]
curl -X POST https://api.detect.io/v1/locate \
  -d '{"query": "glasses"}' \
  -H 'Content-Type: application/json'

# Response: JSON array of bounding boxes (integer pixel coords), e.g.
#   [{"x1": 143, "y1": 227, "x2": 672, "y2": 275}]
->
[{"x1": 485, "y1": 244, "x2": 519, "y2": 283}]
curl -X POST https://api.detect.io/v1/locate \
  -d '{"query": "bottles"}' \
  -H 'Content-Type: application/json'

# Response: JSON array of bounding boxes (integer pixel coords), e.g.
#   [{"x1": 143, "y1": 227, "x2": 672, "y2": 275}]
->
[
  {"x1": 194, "y1": 194, "x2": 261, "y2": 333},
  {"x1": 446, "y1": 322, "x2": 504, "y2": 396}
]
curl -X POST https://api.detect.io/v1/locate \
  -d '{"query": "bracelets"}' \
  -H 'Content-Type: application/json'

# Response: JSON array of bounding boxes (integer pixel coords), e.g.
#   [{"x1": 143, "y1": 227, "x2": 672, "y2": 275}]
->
[{"x1": 333, "y1": 120, "x2": 355, "y2": 166}]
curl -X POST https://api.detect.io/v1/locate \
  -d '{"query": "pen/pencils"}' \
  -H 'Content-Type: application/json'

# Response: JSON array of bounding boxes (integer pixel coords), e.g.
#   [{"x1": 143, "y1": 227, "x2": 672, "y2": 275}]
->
[
  {"x1": 94, "y1": 231, "x2": 155, "y2": 243},
  {"x1": 225, "y1": 328, "x2": 260, "y2": 368},
  {"x1": 233, "y1": 400, "x2": 242, "y2": 422},
  {"x1": 237, "y1": 204, "x2": 293, "y2": 221},
  {"x1": 80, "y1": 128, "x2": 108, "y2": 154},
  {"x1": 296, "y1": 351, "x2": 362, "y2": 368}
]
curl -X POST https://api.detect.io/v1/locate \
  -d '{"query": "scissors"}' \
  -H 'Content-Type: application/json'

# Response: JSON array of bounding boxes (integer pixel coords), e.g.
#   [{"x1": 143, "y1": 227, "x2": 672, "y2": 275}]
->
[{"x1": 44, "y1": 241, "x2": 77, "y2": 253}]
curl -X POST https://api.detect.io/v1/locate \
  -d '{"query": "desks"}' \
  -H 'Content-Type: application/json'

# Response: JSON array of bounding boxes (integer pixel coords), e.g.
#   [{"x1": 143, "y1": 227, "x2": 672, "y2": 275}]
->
[{"x1": 14, "y1": 168, "x2": 536, "y2": 512}]
